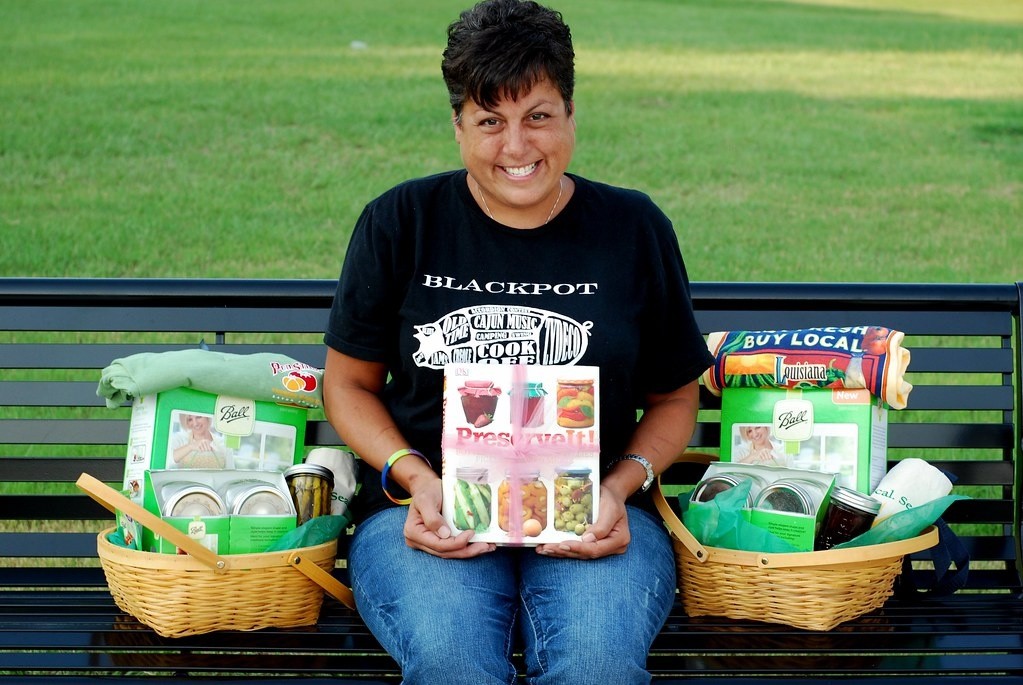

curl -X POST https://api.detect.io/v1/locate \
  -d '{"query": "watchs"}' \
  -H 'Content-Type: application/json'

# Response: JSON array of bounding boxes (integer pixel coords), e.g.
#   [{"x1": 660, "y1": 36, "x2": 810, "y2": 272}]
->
[{"x1": 607, "y1": 453, "x2": 654, "y2": 496}]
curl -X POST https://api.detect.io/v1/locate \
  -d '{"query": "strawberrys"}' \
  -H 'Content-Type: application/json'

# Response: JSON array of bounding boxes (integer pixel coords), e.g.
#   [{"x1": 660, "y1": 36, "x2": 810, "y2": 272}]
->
[{"x1": 474, "y1": 413, "x2": 493, "y2": 428}]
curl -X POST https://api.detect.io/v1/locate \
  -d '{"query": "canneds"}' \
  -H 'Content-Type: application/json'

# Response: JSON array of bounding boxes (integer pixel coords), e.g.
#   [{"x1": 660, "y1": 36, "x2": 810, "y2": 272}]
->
[
  {"x1": 812, "y1": 484, "x2": 881, "y2": 552},
  {"x1": 556, "y1": 378, "x2": 595, "y2": 428},
  {"x1": 453, "y1": 467, "x2": 594, "y2": 536},
  {"x1": 283, "y1": 464, "x2": 334, "y2": 530}
]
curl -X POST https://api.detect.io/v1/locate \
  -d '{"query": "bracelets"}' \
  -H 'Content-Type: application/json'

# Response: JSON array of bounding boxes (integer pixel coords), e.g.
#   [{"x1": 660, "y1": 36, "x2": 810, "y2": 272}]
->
[{"x1": 381, "y1": 449, "x2": 433, "y2": 504}]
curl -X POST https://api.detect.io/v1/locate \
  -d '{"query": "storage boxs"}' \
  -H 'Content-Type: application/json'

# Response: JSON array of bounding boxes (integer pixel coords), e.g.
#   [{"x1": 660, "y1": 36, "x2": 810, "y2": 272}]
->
[
  {"x1": 114, "y1": 382, "x2": 308, "y2": 554},
  {"x1": 689, "y1": 387, "x2": 888, "y2": 551},
  {"x1": 442, "y1": 362, "x2": 603, "y2": 549}
]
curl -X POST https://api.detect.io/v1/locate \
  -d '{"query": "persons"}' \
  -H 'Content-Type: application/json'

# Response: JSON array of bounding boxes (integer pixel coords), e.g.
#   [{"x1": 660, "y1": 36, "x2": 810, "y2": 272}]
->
[
  {"x1": 131, "y1": 481, "x2": 141, "y2": 497},
  {"x1": 323, "y1": 0, "x2": 717, "y2": 685},
  {"x1": 731, "y1": 424, "x2": 795, "y2": 468},
  {"x1": 166, "y1": 412, "x2": 235, "y2": 470}
]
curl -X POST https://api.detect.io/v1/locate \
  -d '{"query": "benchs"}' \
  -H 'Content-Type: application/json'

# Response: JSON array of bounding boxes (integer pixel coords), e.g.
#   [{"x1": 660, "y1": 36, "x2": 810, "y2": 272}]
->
[{"x1": 0, "y1": 277, "x2": 1023, "y2": 685}]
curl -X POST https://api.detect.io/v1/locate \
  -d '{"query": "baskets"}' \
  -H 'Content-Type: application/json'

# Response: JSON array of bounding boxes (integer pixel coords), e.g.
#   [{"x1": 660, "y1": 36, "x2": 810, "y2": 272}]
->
[
  {"x1": 75, "y1": 472, "x2": 356, "y2": 639},
  {"x1": 651, "y1": 452, "x2": 940, "y2": 631}
]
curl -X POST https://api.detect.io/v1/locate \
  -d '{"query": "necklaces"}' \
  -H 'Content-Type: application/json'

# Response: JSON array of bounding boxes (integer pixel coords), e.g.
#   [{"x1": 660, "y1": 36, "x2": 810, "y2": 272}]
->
[{"x1": 480, "y1": 179, "x2": 563, "y2": 230}]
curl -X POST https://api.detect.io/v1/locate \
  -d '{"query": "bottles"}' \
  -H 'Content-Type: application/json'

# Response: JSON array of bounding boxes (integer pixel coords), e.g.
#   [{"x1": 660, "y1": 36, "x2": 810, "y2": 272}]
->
[
  {"x1": 497, "y1": 471, "x2": 548, "y2": 535},
  {"x1": 556, "y1": 377, "x2": 596, "y2": 428},
  {"x1": 283, "y1": 464, "x2": 333, "y2": 529},
  {"x1": 814, "y1": 485, "x2": 882, "y2": 553},
  {"x1": 553, "y1": 467, "x2": 593, "y2": 533},
  {"x1": 449, "y1": 467, "x2": 492, "y2": 533}
]
